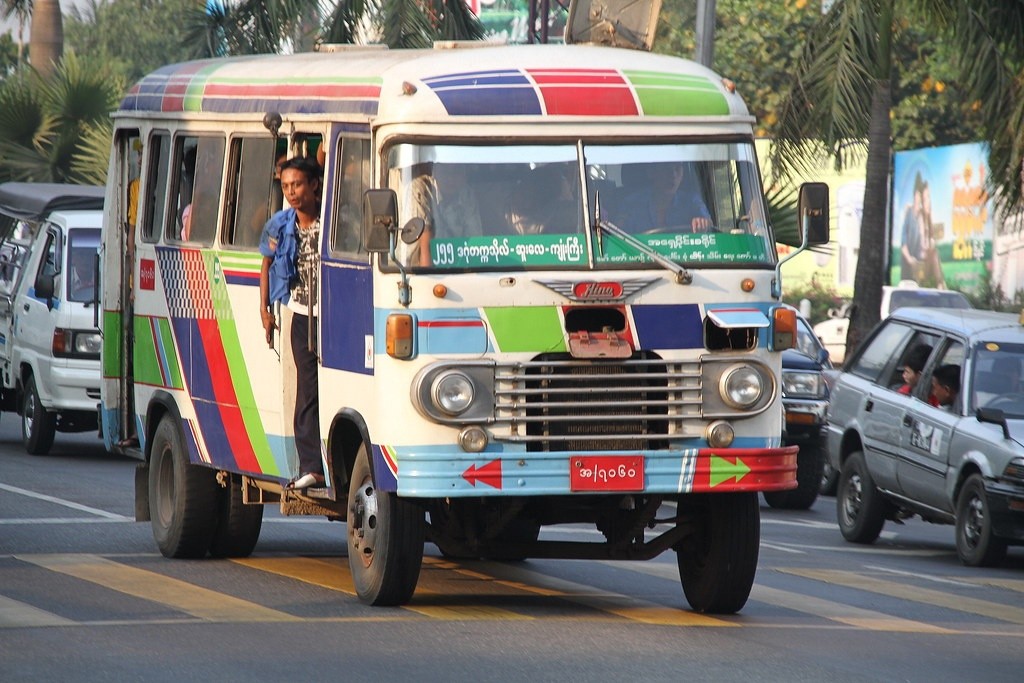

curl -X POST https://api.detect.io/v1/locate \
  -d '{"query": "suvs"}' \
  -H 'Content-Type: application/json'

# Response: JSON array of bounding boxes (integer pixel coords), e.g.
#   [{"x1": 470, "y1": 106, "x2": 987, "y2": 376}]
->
[
  {"x1": 762, "y1": 302, "x2": 830, "y2": 512},
  {"x1": 832, "y1": 304, "x2": 1024, "y2": 567}
]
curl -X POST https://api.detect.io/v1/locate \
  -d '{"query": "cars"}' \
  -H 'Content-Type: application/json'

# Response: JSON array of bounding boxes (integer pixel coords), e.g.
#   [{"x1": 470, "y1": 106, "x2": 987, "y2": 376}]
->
[{"x1": 814, "y1": 280, "x2": 976, "y2": 364}]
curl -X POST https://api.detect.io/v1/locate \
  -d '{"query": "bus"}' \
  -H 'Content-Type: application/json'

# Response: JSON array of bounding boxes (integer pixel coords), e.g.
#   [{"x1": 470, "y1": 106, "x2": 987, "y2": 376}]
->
[{"x1": 97, "y1": 37, "x2": 829, "y2": 614}]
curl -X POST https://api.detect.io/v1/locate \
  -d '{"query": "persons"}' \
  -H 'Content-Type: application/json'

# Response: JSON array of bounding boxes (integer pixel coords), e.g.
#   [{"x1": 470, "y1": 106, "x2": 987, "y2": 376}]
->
[
  {"x1": 619, "y1": 162, "x2": 713, "y2": 233},
  {"x1": 896, "y1": 344, "x2": 933, "y2": 397},
  {"x1": 932, "y1": 364, "x2": 961, "y2": 409},
  {"x1": 260, "y1": 156, "x2": 326, "y2": 490},
  {"x1": 991, "y1": 358, "x2": 1024, "y2": 393},
  {"x1": 118, "y1": 137, "x2": 143, "y2": 448},
  {"x1": 900, "y1": 169, "x2": 948, "y2": 289},
  {"x1": 180, "y1": 138, "x2": 579, "y2": 270}
]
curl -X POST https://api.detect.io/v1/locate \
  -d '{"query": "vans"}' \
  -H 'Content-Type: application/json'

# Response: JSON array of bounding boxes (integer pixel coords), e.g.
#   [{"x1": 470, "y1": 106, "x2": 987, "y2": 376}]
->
[{"x1": 781, "y1": 302, "x2": 835, "y2": 372}]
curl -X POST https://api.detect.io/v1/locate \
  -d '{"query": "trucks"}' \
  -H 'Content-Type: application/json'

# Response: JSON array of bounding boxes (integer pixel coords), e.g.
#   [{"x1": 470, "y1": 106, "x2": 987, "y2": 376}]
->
[{"x1": 1, "y1": 182, "x2": 105, "y2": 450}]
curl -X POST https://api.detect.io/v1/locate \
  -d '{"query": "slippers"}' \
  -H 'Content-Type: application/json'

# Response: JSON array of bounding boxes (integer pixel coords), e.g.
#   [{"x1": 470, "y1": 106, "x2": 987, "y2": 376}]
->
[{"x1": 282, "y1": 473, "x2": 326, "y2": 491}]
